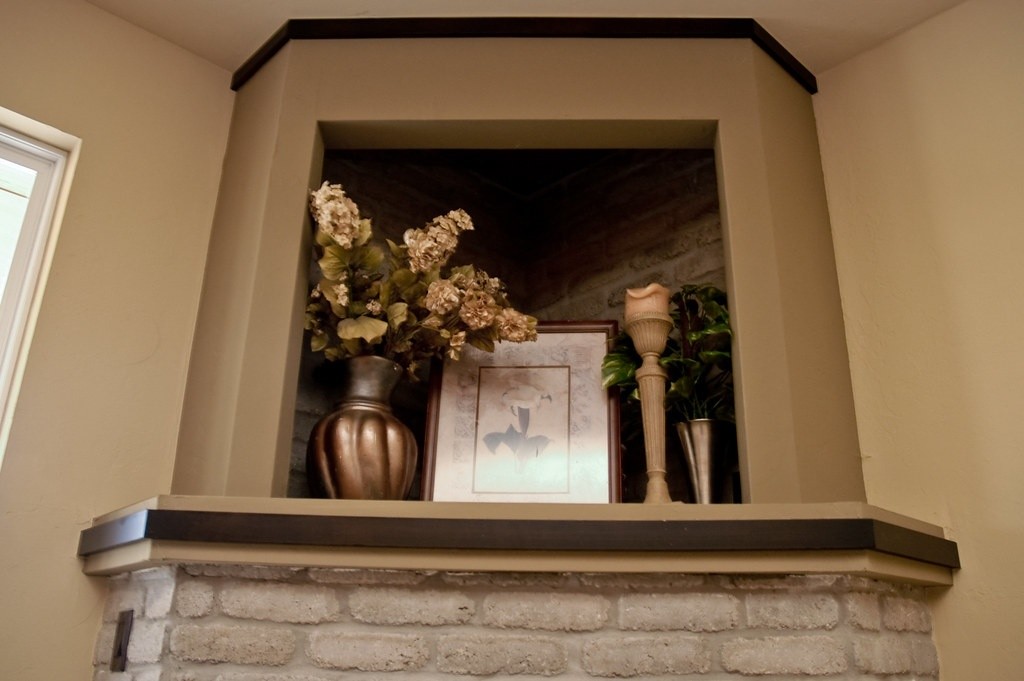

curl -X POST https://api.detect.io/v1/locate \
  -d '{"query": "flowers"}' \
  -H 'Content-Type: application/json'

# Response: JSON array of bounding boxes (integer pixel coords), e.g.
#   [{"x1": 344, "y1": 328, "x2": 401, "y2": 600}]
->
[{"x1": 299, "y1": 182, "x2": 540, "y2": 385}]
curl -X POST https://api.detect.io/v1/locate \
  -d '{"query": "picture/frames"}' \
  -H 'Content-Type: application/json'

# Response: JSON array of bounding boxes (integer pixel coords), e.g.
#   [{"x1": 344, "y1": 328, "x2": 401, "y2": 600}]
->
[{"x1": 420, "y1": 312, "x2": 625, "y2": 503}]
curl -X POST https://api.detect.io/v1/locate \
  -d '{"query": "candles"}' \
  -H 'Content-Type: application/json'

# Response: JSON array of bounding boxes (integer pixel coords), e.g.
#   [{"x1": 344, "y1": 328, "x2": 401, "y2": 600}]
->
[{"x1": 619, "y1": 280, "x2": 674, "y2": 324}]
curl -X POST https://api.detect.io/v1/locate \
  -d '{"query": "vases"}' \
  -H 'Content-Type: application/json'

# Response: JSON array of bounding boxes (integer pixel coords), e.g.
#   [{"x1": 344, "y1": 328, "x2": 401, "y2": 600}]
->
[{"x1": 303, "y1": 354, "x2": 427, "y2": 497}]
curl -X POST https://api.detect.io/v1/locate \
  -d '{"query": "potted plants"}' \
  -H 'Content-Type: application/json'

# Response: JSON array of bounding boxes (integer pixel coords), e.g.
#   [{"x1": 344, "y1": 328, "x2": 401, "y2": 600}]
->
[{"x1": 597, "y1": 276, "x2": 740, "y2": 504}]
